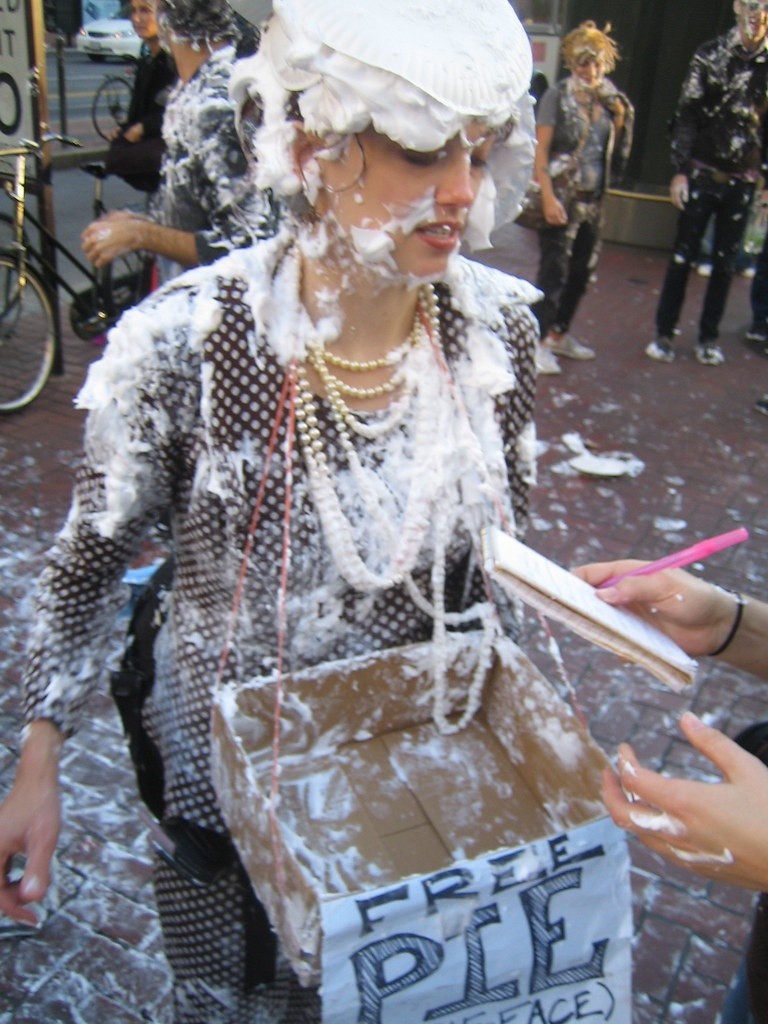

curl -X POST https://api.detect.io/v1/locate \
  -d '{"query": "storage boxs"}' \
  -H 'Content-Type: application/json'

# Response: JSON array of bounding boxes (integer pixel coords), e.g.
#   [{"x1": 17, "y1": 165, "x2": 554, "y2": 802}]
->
[{"x1": 209, "y1": 630, "x2": 632, "y2": 1024}]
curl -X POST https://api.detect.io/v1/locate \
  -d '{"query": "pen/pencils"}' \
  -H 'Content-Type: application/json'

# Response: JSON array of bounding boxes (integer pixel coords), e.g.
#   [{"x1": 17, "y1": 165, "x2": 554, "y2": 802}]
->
[{"x1": 594, "y1": 525, "x2": 749, "y2": 589}]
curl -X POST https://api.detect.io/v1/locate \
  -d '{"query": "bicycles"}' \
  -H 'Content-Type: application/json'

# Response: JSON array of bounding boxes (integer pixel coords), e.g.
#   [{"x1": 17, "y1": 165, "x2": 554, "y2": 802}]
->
[{"x1": 0, "y1": 120, "x2": 143, "y2": 416}]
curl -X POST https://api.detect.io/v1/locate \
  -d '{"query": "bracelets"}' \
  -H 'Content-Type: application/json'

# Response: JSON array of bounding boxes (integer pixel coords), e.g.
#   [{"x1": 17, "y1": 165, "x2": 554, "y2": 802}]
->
[{"x1": 706, "y1": 582, "x2": 744, "y2": 657}]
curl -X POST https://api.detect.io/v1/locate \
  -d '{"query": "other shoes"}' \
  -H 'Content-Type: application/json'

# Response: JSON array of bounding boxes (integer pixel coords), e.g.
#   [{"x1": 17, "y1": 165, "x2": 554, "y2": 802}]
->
[
  {"x1": 540, "y1": 334, "x2": 594, "y2": 360},
  {"x1": 755, "y1": 400, "x2": 768, "y2": 416},
  {"x1": 648, "y1": 335, "x2": 674, "y2": 362},
  {"x1": 693, "y1": 331, "x2": 720, "y2": 364},
  {"x1": 534, "y1": 346, "x2": 560, "y2": 374},
  {"x1": 747, "y1": 320, "x2": 767, "y2": 340}
]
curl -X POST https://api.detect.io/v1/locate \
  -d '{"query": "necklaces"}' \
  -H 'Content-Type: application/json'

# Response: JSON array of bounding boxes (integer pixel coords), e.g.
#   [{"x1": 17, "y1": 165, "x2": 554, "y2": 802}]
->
[{"x1": 274, "y1": 245, "x2": 499, "y2": 736}]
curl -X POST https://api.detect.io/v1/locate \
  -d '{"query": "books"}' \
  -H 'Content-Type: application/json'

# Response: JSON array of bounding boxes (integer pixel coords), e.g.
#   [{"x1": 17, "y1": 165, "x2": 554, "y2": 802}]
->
[{"x1": 481, "y1": 524, "x2": 697, "y2": 693}]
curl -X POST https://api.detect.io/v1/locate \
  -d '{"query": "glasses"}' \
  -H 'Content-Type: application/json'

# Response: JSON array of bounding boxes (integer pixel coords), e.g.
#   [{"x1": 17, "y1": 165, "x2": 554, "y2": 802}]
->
[{"x1": 742, "y1": 0, "x2": 768, "y2": 12}]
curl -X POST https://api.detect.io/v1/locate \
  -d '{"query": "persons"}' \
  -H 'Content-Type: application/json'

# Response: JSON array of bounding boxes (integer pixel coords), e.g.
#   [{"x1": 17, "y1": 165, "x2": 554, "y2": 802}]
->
[
  {"x1": 526, "y1": 23, "x2": 624, "y2": 376},
  {"x1": 645, "y1": 0, "x2": 767, "y2": 418},
  {"x1": 565, "y1": 558, "x2": 768, "y2": 1024},
  {"x1": 0, "y1": 0, "x2": 538, "y2": 1024},
  {"x1": 78, "y1": 0, "x2": 287, "y2": 632}
]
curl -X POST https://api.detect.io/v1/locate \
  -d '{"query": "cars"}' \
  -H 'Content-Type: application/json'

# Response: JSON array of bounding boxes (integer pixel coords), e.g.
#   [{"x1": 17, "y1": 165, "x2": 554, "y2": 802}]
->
[{"x1": 75, "y1": 4, "x2": 148, "y2": 65}]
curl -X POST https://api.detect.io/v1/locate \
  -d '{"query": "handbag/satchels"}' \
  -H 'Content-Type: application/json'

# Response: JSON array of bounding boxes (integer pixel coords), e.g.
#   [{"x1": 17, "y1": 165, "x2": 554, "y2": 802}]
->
[
  {"x1": 106, "y1": 130, "x2": 161, "y2": 191},
  {"x1": 515, "y1": 160, "x2": 576, "y2": 231}
]
